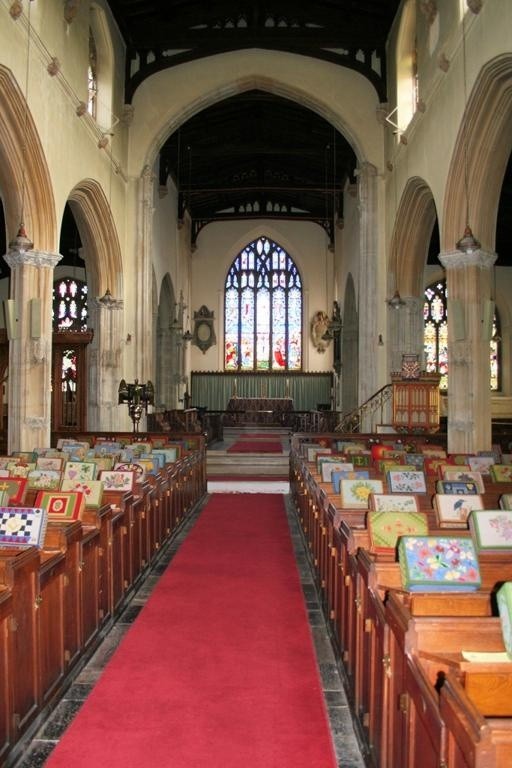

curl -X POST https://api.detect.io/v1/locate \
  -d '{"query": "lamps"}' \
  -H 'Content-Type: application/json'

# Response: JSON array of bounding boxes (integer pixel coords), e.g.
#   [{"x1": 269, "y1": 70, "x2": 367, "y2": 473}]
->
[
  {"x1": 455, "y1": 19, "x2": 482, "y2": 253},
  {"x1": 8, "y1": 0, "x2": 34, "y2": 255},
  {"x1": 387, "y1": 163, "x2": 406, "y2": 310},
  {"x1": 99, "y1": 146, "x2": 117, "y2": 309}
]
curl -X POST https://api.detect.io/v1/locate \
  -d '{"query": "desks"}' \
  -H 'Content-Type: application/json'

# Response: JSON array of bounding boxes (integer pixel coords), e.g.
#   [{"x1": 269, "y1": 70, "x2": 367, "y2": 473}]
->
[
  {"x1": 0, "y1": 437, "x2": 206, "y2": 764},
  {"x1": 287, "y1": 441, "x2": 512, "y2": 768}
]
